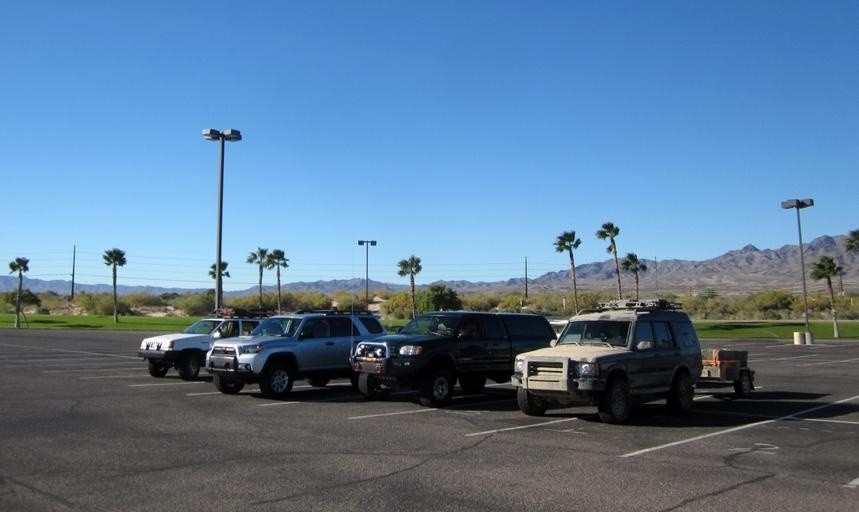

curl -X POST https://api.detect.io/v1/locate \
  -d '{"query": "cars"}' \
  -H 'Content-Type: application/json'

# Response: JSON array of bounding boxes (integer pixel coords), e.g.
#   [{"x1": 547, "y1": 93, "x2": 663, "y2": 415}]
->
[{"x1": 543, "y1": 316, "x2": 573, "y2": 342}]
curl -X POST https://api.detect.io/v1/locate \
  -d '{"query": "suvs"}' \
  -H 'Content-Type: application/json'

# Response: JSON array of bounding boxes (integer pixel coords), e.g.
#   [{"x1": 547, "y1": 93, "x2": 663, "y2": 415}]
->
[
  {"x1": 205, "y1": 311, "x2": 387, "y2": 399},
  {"x1": 137, "y1": 307, "x2": 287, "y2": 381},
  {"x1": 511, "y1": 299, "x2": 702, "y2": 423},
  {"x1": 349, "y1": 310, "x2": 558, "y2": 408}
]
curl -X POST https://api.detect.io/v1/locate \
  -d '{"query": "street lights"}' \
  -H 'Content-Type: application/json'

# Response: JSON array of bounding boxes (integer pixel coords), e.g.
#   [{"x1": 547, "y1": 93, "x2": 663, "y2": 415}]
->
[
  {"x1": 357, "y1": 240, "x2": 377, "y2": 313},
  {"x1": 781, "y1": 198, "x2": 814, "y2": 345},
  {"x1": 202, "y1": 127, "x2": 243, "y2": 317}
]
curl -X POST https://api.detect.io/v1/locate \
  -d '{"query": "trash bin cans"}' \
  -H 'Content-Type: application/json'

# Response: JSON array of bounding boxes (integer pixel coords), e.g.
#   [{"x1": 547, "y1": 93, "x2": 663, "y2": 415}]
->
[{"x1": 793, "y1": 332, "x2": 805, "y2": 345}]
